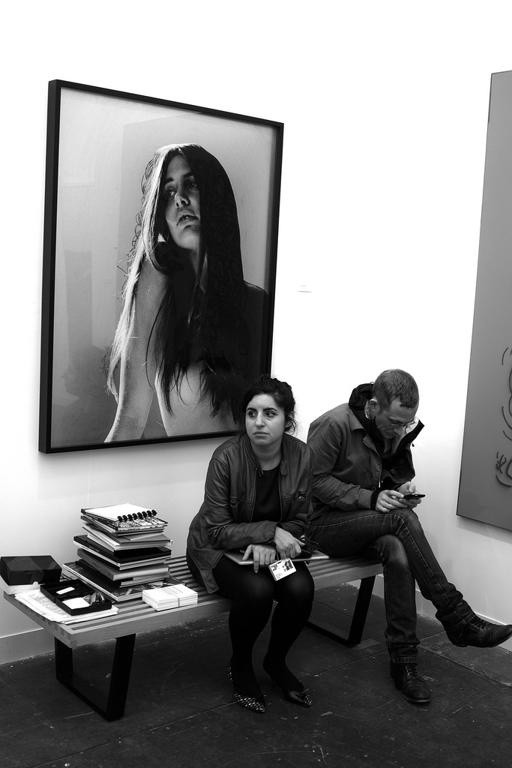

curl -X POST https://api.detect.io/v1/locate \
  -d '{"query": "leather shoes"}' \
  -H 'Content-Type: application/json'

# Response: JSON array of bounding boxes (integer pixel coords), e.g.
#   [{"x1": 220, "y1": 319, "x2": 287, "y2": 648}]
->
[
  {"x1": 226, "y1": 661, "x2": 265, "y2": 713},
  {"x1": 390, "y1": 660, "x2": 432, "y2": 704},
  {"x1": 447, "y1": 612, "x2": 512, "y2": 649},
  {"x1": 279, "y1": 680, "x2": 313, "y2": 708}
]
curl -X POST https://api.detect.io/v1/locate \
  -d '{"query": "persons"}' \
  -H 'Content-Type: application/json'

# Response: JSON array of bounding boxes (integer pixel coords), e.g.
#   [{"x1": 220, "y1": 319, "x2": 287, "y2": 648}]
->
[
  {"x1": 187, "y1": 376, "x2": 315, "y2": 714},
  {"x1": 304, "y1": 367, "x2": 510, "y2": 704},
  {"x1": 101, "y1": 139, "x2": 268, "y2": 435}
]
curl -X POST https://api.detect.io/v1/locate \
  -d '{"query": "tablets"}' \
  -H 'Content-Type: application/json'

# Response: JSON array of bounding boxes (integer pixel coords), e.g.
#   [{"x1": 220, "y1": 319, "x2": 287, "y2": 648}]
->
[{"x1": 223, "y1": 549, "x2": 330, "y2": 565}]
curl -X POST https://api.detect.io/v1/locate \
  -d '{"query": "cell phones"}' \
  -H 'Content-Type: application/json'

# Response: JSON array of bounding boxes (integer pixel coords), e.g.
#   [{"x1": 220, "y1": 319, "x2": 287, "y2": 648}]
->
[{"x1": 403, "y1": 494, "x2": 425, "y2": 499}]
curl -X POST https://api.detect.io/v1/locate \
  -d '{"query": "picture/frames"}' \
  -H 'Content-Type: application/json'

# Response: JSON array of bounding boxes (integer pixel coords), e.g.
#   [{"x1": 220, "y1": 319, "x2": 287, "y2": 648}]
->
[{"x1": 36, "y1": 75, "x2": 287, "y2": 455}]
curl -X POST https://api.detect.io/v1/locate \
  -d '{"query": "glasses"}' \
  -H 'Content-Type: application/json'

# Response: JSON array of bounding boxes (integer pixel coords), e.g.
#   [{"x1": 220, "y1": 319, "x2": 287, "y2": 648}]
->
[{"x1": 388, "y1": 419, "x2": 416, "y2": 429}]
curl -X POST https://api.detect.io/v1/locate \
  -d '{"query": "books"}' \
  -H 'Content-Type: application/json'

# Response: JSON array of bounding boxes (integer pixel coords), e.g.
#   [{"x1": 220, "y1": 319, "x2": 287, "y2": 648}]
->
[{"x1": 63, "y1": 500, "x2": 202, "y2": 613}]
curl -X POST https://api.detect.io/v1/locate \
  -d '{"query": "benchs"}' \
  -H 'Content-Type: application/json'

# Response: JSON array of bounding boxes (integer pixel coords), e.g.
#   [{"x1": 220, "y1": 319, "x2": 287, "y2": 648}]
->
[{"x1": 3, "y1": 555, "x2": 381, "y2": 718}]
